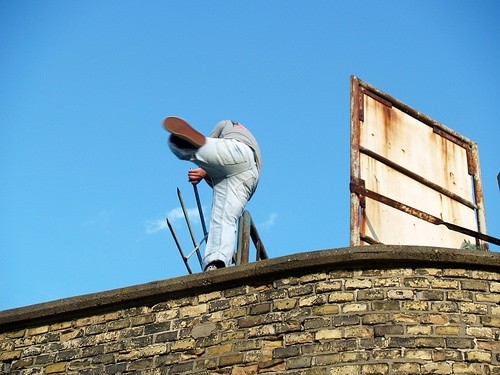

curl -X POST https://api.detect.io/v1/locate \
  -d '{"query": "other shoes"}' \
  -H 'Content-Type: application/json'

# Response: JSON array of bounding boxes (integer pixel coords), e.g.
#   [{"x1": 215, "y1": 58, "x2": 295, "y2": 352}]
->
[
  {"x1": 203, "y1": 262, "x2": 219, "y2": 271},
  {"x1": 163, "y1": 117, "x2": 205, "y2": 148}
]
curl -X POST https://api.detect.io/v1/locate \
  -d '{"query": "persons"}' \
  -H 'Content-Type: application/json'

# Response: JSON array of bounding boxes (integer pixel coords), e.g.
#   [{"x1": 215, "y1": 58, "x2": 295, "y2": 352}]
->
[{"x1": 163, "y1": 117, "x2": 260, "y2": 270}]
